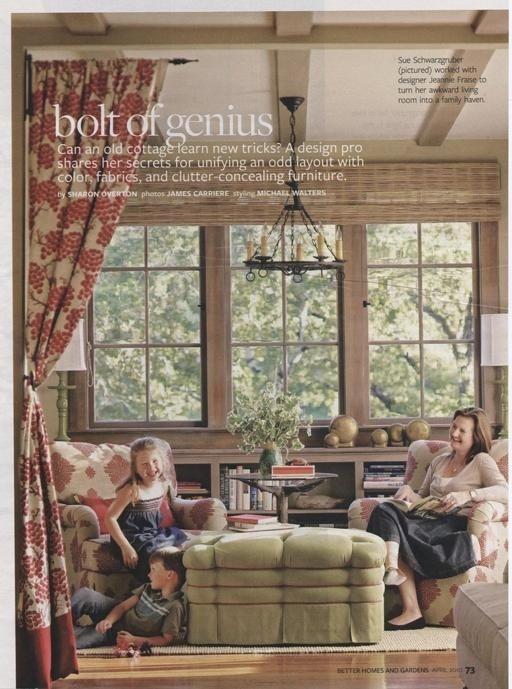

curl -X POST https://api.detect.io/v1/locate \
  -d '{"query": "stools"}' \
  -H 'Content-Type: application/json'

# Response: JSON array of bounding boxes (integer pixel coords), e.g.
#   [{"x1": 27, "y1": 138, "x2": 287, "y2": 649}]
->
[{"x1": 451, "y1": 585, "x2": 508, "y2": 688}]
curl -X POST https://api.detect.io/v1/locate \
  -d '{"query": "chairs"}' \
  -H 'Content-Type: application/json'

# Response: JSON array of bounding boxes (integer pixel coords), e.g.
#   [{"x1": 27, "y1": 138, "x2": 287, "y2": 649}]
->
[
  {"x1": 49, "y1": 442, "x2": 227, "y2": 626},
  {"x1": 348, "y1": 440, "x2": 508, "y2": 627}
]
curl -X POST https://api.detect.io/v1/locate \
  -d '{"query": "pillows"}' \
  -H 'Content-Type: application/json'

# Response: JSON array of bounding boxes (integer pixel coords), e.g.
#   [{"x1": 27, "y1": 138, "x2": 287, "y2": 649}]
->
[{"x1": 75, "y1": 495, "x2": 176, "y2": 534}]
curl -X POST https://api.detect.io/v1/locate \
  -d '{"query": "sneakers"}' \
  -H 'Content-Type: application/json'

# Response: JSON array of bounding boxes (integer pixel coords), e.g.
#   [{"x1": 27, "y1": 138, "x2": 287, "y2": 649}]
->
[
  {"x1": 384, "y1": 567, "x2": 407, "y2": 587},
  {"x1": 384, "y1": 617, "x2": 425, "y2": 631}
]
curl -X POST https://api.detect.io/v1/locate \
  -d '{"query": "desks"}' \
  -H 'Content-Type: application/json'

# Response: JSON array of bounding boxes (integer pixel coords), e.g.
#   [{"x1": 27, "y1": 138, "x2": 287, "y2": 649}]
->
[{"x1": 226, "y1": 473, "x2": 338, "y2": 523}]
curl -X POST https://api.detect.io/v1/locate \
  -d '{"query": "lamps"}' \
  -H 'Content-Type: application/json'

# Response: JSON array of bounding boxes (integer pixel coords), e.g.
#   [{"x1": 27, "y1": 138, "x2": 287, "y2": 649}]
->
[
  {"x1": 479, "y1": 313, "x2": 508, "y2": 438},
  {"x1": 243, "y1": 97, "x2": 348, "y2": 283},
  {"x1": 48, "y1": 318, "x2": 87, "y2": 441}
]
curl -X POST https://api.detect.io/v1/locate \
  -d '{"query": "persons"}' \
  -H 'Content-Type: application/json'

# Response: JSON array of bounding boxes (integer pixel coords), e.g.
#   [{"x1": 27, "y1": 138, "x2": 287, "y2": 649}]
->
[
  {"x1": 365, "y1": 405, "x2": 508, "y2": 632},
  {"x1": 69, "y1": 546, "x2": 187, "y2": 649},
  {"x1": 107, "y1": 435, "x2": 187, "y2": 587}
]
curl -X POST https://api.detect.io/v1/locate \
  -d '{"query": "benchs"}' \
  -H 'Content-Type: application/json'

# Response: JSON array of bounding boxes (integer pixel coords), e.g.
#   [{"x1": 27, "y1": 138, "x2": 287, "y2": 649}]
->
[{"x1": 182, "y1": 526, "x2": 387, "y2": 646}]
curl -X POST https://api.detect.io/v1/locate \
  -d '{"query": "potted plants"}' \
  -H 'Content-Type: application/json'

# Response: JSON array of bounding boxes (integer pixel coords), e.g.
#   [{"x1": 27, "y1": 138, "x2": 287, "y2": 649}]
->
[{"x1": 226, "y1": 382, "x2": 314, "y2": 476}]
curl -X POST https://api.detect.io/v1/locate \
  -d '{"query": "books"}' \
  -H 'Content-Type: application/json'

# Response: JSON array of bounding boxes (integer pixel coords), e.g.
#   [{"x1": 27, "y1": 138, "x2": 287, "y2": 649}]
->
[
  {"x1": 362, "y1": 462, "x2": 408, "y2": 489},
  {"x1": 269, "y1": 464, "x2": 315, "y2": 479},
  {"x1": 220, "y1": 463, "x2": 279, "y2": 511},
  {"x1": 226, "y1": 513, "x2": 282, "y2": 529},
  {"x1": 383, "y1": 494, "x2": 462, "y2": 519}
]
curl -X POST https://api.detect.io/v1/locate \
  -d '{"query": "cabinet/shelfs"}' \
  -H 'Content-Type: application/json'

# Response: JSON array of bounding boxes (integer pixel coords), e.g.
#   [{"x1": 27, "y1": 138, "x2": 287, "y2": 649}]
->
[{"x1": 174, "y1": 453, "x2": 405, "y2": 524}]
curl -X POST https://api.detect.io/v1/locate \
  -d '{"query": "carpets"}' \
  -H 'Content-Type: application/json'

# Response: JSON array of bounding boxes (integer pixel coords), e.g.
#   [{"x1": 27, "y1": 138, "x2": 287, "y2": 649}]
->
[{"x1": 75, "y1": 626, "x2": 460, "y2": 655}]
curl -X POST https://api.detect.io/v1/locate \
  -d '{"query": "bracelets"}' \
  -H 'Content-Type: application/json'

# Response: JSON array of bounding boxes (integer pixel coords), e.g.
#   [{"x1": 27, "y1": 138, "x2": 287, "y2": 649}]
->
[{"x1": 469, "y1": 489, "x2": 479, "y2": 503}]
